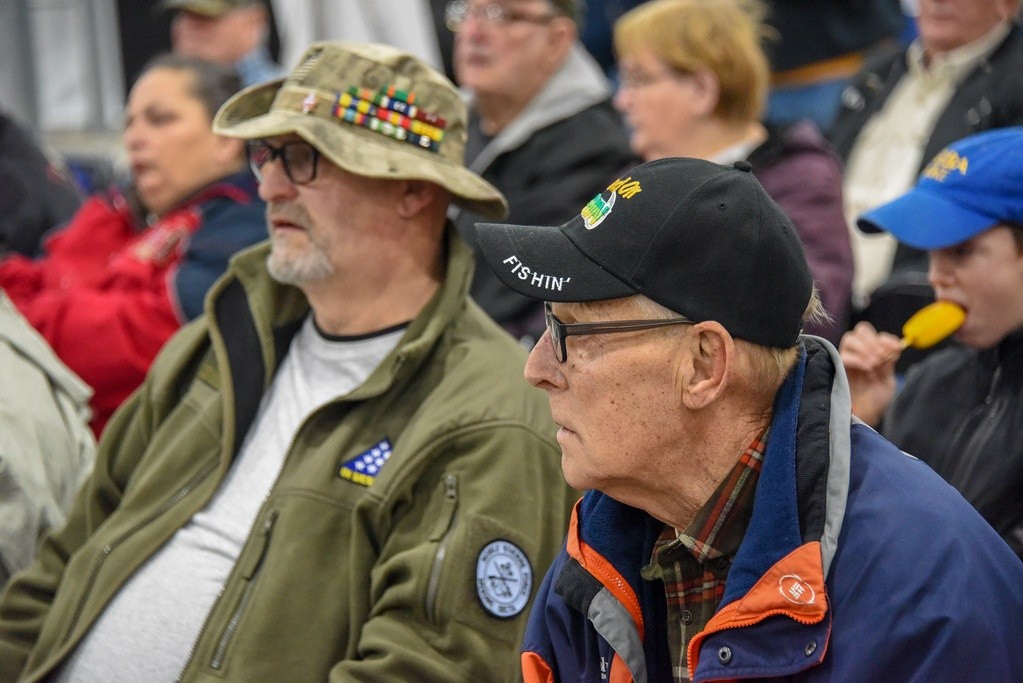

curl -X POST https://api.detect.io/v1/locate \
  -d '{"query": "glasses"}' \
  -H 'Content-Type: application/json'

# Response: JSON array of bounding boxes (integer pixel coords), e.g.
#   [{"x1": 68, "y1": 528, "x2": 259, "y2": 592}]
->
[
  {"x1": 444, "y1": 0, "x2": 556, "y2": 32},
  {"x1": 542, "y1": 301, "x2": 700, "y2": 364},
  {"x1": 245, "y1": 136, "x2": 323, "y2": 184}
]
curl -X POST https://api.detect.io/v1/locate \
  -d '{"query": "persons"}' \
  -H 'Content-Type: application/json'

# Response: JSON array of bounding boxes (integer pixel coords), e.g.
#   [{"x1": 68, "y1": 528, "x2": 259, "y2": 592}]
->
[
  {"x1": 826, "y1": 0, "x2": 1022, "y2": 381},
  {"x1": 611, "y1": 1, "x2": 856, "y2": 349},
  {"x1": 836, "y1": 125, "x2": 1021, "y2": 560},
  {"x1": 153, "y1": 1, "x2": 285, "y2": 94},
  {"x1": 0, "y1": 55, "x2": 275, "y2": 450},
  {"x1": 0, "y1": 37, "x2": 568, "y2": 683},
  {"x1": 475, "y1": 158, "x2": 1021, "y2": 682},
  {"x1": 428, "y1": 0, "x2": 643, "y2": 353}
]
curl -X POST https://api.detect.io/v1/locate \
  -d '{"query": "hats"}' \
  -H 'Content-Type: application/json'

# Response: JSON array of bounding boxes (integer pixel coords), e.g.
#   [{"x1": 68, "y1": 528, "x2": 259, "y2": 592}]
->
[
  {"x1": 472, "y1": 156, "x2": 813, "y2": 353},
  {"x1": 856, "y1": 125, "x2": 1023, "y2": 251},
  {"x1": 210, "y1": 39, "x2": 510, "y2": 221}
]
